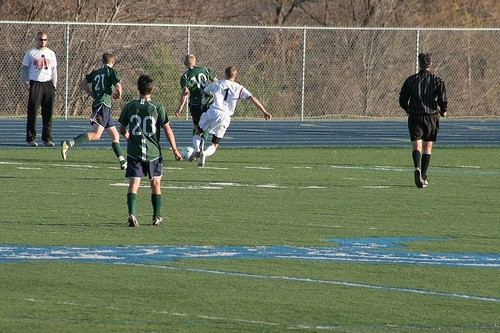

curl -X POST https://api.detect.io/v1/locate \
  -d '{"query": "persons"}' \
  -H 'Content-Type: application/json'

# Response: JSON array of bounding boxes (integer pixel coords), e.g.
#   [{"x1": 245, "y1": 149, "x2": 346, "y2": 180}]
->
[
  {"x1": 20, "y1": 31, "x2": 57, "y2": 147},
  {"x1": 189, "y1": 65, "x2": 273, "y2": 168},
  {"x1": 174, "y1": 54, "x2": 219, "y2": 157},
  {"x1": 116, "y1": 74, "x2": 182, "y2": 227},
  {"x1": 399, "y1": 53, "x2": 448, "y2": 188},
  {"x1": 61, "y1": 52, "x2": 127, "y2": 170}
]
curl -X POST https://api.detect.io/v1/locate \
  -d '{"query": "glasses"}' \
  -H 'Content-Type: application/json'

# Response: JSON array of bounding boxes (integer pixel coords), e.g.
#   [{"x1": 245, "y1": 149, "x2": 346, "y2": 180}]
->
[{"x1": 39, "y1": 39, "x2": 47, "y2": 41}]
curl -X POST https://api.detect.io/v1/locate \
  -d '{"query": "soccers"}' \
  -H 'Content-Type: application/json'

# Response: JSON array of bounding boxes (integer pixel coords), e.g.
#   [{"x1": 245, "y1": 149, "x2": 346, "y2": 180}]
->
[{"x1": 181, "y1": 143, "x2": 196, "y2": 161}]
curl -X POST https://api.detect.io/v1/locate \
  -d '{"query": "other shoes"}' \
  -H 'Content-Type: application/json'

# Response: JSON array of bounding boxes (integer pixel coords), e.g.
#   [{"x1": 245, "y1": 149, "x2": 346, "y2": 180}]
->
[
  {"x1": 421, "y1": 179, "x2": 429, "y2": 186},
  {"x1": 43, "y1": 141, "x2": 57, "y2": 148},
  {"x1": 128, "y1": 215, "x2": 146, "y2": 228},
  {"x1": 197, "y1": 151, "x2": 207, "y2": 167},
  {"x1": 414, "y1": 167, "x2": 424, "y2": 189},
  {"x1": 61, "y1": 140, "x2": 72, "y2": 160},
  {"x1": 152, "y1": 215, "x2": 163, "y2": 226},
  {"x1": 27, "y1": 141, "x2": 38, "y2": 148},
  {"x1": 189, "y1": 149, "x2": 200, "y2": 162},
  {"x1": 121, "y1": 162, "x2": 128, "y2": 170}
]
